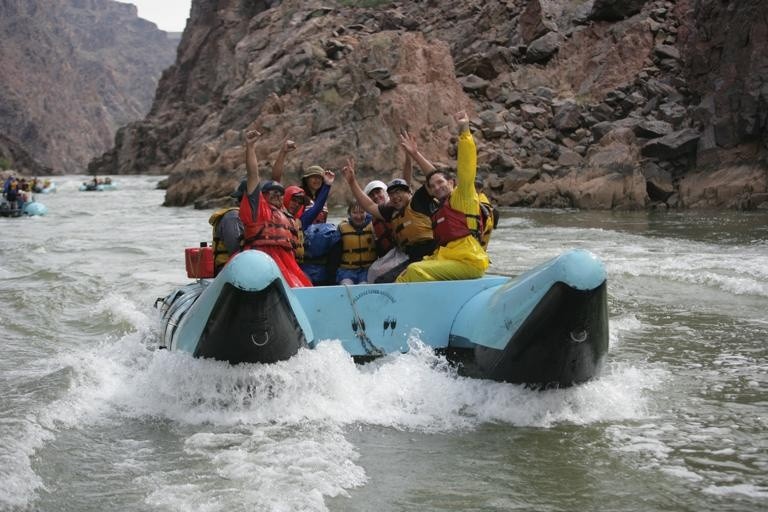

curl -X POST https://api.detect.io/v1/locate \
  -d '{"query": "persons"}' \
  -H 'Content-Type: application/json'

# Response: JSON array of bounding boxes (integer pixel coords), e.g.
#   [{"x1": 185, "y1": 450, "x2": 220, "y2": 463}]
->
[
  {"x1": 338, "y1": 110, "x2": 488, "y2": 284},
  {"x1": 208, "y1": 130, "x2": 335, "y2": 288},
  {"x1": 83, "y1": 175, "x2": 112, "y2": 190},
  {"x1": 0, "y1": 173, "x2": 51, "y2": 210}
]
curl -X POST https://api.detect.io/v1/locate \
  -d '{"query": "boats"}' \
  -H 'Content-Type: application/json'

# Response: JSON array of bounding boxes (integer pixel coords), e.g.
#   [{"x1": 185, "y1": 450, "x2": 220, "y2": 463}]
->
[
  {"x1": 159, "y1": 249, "x2": 608, "y2": 410},
  {"x1": 9, "y1": 175, "x2": 117, "y2": 216}
]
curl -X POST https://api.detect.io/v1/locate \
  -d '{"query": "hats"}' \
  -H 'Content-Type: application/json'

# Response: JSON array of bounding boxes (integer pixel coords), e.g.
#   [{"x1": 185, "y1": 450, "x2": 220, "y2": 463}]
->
[
  {"x1": 262, "y1": 180, "x2": 286, "y2": 195},
  {"x1": 294, "y1": 191, "x2": 311, "y2": 206},
  {"x1": 231, "y1": 179, "x2": 247, "y2": 198},
  {"x1": 300, "y1": 166, "x2": 324, "y2": 180},
  {"x1": 386, "y1": 178, "x2": 410, "y2": 193}
]
